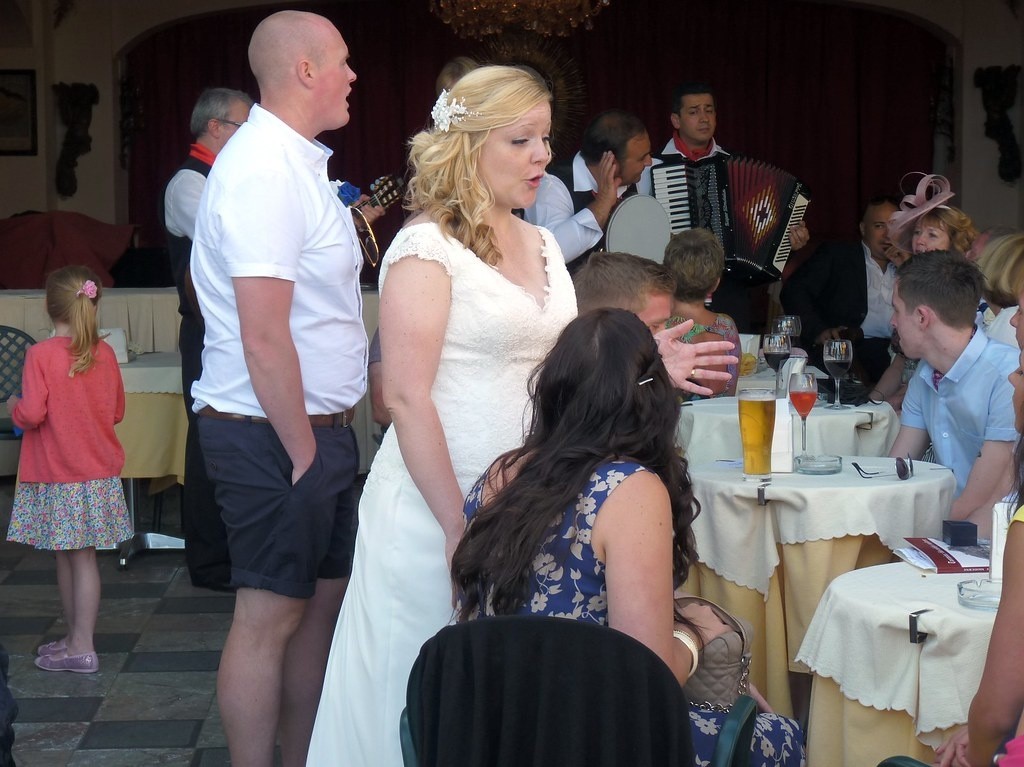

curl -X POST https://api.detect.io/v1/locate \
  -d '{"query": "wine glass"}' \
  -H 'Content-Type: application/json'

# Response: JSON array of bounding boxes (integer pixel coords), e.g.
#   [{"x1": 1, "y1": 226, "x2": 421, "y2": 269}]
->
[
  {"x1": 789, "y1": 373, "x2": 818, "y2": 458},
  {"x1": 763, "y1": 333, "x2": 792, "y2": 399},
  {"x1": 822, "y1": 339, "x2": 853, "y2": 410}
]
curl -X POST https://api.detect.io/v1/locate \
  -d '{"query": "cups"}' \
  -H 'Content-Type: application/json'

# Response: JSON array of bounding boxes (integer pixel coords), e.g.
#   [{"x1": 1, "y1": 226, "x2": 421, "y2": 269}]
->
[
  {"x1": 773, "y1": 316, "x2": 802, "y2": 349},
  {"x1": 736, "y1": 376, "x2": 777, "y2": 483}
]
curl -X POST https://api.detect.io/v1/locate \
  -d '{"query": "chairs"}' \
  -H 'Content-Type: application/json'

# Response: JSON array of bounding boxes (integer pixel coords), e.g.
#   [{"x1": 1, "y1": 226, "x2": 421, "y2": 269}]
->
[
  {"x1": 396, "y1": 617, "x2": 758, "y2": 767},
  {"x1": 0, "y1": 324, "x2": 40, "y2": 440}
]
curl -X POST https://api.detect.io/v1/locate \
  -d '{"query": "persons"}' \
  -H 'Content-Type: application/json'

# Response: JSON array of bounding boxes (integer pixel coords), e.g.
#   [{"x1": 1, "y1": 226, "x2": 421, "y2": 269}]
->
[
  {"x1": 190, "y1": 10, "x2": 368, "y2": 767},
  {"x1": 888, "y1": 248, "x2": 1021, "y2": 538},
  {"x1": 157, "y1": 88, "x2": 385, "y2": 593},
  {"x1": 307, "y1": 65, "x2": 737, "y2": 767},
  {"x1": 452, "y1": 308, "x2": 806, "y2": 767},
  {"x1": 437, "y1": 55, "x2": 995, "y2": 415},
  {"x1": 936, "y1": 289, "x2": 1024, "y2": 767},
  {"x1": 6, "y1": 265, "x2": 133, "y2": 673}
]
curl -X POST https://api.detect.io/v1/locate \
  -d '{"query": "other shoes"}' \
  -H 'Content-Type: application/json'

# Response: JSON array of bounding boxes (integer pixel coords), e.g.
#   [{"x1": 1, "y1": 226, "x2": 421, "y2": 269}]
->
[{"x1": 34, "y1": 638, "x2": 100, "y2": 674}]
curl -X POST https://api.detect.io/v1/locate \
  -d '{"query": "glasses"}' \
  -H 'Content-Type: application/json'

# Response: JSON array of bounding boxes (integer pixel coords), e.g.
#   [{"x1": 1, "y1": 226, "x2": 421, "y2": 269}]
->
[
  {"x1": 852, "y1": 452, "x2": 914, "y2": 480},
  {"x1": 348, "y1": 205, "x2": 379, "y2": 267},
  {"x1": 872, "y1": 196, "x2": 899, "y2": 205}
]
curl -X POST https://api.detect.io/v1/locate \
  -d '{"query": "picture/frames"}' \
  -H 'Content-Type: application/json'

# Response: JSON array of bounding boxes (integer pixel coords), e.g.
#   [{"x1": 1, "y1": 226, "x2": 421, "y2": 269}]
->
[{"x1": 0, "y1": 69, "x2": 38, "y2": 155}]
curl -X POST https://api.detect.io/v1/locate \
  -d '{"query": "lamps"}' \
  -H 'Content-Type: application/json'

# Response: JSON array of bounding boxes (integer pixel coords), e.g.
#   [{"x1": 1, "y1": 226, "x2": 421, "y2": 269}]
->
[{"x1": 429, "y1": 0, "x2": 610, "y2": 41}]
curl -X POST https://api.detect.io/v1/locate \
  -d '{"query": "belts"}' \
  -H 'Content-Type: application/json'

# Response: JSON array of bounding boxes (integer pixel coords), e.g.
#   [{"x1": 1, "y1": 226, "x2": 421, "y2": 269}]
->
[{"x1": 196, "y1": 404, "x2": 356, "y2": 429}]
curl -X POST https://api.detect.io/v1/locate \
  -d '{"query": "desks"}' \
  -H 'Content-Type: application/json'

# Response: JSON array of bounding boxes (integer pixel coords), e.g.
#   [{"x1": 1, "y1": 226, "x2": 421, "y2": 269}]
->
[
  {"x1": 794, "y1": 545, "x2": 1002, "y2": 767},
  {"x1": 685, "y1": 455, "x2": 955, "y2": 719},
  {"x1": 113, "y1": 351, "x2": 189, "y2": 571},
  {"x1": 674, "y1": 395, "x2": 901, "y2": 461}
]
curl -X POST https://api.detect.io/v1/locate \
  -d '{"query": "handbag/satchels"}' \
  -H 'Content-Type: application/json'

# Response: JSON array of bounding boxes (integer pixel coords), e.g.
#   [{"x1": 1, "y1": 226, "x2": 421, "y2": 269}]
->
[{"x1": 674, "y1": 596, "x2": 751, "y2": 711}]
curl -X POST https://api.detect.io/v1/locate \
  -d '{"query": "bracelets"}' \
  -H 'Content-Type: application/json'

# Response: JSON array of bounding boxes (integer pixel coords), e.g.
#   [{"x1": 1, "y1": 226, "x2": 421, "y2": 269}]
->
[{"x1": 673, "y1": 629, "x2": 698, "y2": 678}]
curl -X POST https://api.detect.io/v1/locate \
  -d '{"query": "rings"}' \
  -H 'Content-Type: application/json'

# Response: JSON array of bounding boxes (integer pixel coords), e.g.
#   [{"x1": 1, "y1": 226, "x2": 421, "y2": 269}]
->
[{"x1": 691, "y1": 369, "x2": 696, "y2": 379}]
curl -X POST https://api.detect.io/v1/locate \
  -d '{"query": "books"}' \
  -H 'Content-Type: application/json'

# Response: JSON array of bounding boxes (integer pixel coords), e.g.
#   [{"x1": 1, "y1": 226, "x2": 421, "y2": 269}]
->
[{"x1": 892, "y1": 537, "x2": 991, "y2": 574}]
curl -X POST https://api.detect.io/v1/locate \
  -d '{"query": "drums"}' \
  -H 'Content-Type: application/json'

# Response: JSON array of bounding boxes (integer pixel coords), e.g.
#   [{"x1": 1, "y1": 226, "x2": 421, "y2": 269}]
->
[{"x1": 606, "y1": 193, "x2": 673, "y2": 265}]
